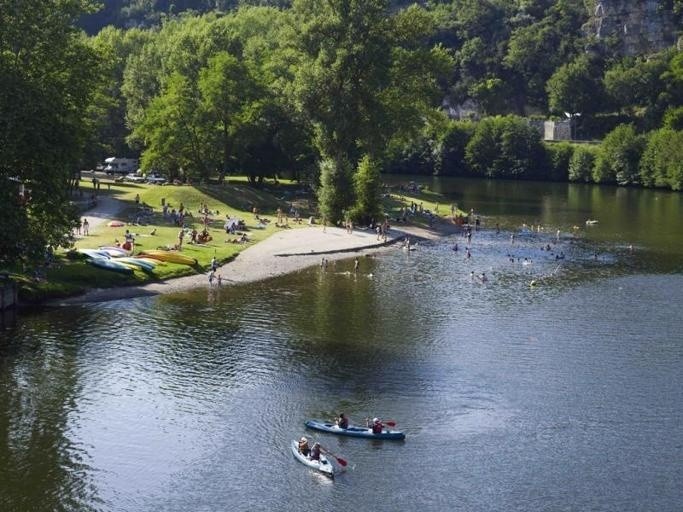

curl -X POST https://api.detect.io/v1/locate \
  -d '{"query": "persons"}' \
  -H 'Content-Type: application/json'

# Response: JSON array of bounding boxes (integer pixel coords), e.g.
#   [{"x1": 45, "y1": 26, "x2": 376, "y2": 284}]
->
[
  {"x1": 366, "y1": 417, "x2": 383, "y2": 434},
  {"x1": 205, "y1": 288, "x2": 225, "y2": 302},
  {"x1": 308, "y1": 442, "x2": 329, "y2": 464},
  {"x1": 296, "y1": 436, "x2": 311, "y2": 458},
  {"x1": 52, "y1": 175, "x2": 616, "y2": 287},
  {"x1": 334, "y1": 412, "x2": 347, "y2": 429}
]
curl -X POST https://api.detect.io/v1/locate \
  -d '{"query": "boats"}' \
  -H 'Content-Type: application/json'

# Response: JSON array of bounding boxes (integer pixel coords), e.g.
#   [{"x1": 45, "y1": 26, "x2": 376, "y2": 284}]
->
[
  {"x1": 304, "y1": 418, "x2": 406, "y2": 445},
  {"x1": 288, "y1": 439, "x2": 345, "y2": 480},
  {"x1": 69, "y1": 243, "x2": 198, "y2": 275}
]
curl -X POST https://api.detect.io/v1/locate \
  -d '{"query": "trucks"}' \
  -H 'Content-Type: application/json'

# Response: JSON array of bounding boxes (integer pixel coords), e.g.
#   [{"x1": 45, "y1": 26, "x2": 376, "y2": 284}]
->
[{"x1": 103, "y1": 158, "x2": 139, "y2": 174}]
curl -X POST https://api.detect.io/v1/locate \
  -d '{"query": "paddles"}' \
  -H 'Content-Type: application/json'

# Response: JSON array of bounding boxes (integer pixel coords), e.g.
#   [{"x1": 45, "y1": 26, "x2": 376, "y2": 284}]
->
[
  {"x1": 323, "y1": 448, "x2": 347, "y2": 466},
  {"x1": 381, "y1": 422, "x2": 395, "y2": 426}
]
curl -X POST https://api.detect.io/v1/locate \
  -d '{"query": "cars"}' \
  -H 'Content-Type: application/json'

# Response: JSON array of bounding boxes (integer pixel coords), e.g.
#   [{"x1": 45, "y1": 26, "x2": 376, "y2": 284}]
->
[
  {"x1": 125, "y1": 172, "x2": 143, "y2": 183},
  {"x1": 146, "y1": 173, "x2": 166, "y2": 183}
]
